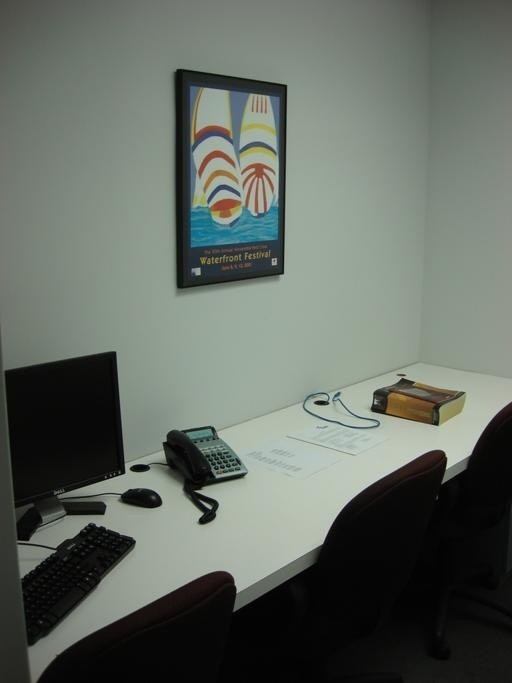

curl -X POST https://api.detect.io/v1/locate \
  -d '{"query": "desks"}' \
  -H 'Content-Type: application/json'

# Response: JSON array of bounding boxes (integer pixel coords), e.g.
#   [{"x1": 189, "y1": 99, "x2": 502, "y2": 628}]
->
[{"x1": 15, "y1": 361, "x2": 512, "y2": 683}]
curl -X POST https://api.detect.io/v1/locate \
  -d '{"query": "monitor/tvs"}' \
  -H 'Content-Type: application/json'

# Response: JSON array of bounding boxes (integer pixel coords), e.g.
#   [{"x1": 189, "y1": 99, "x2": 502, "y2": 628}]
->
[{"x1": 4, "y1": 349, "x2": 127, "y2": 542}]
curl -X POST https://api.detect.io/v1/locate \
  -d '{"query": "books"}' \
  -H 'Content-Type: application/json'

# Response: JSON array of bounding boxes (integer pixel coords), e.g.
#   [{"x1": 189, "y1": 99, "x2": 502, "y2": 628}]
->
[{"x1": 370, "y1": 376, "x2": 467, "y2": 427}]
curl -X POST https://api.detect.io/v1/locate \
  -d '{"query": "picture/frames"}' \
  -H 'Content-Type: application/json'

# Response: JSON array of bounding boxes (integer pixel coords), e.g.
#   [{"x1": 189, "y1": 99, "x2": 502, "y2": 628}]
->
[{"x1": 174, "y1": 68, "x2": 286, "y2": 289}]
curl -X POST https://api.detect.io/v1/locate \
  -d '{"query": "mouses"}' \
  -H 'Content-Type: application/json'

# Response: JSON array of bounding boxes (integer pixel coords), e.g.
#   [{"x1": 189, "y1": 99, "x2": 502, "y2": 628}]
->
[{"x1": 119, "y1": 487, "x2": 163, "y2": 508}]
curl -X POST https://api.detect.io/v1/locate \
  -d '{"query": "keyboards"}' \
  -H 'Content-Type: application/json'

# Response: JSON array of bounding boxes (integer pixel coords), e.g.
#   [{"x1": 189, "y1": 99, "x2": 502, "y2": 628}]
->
[{"x1": 15, "y1": 521, "x2": 138, "y2": 645}]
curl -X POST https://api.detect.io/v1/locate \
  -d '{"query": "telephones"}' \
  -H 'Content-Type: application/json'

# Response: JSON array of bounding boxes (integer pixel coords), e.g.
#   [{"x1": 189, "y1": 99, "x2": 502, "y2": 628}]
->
[{"x1": 162, "y1": 425, "x2": 247, "y2": 485}]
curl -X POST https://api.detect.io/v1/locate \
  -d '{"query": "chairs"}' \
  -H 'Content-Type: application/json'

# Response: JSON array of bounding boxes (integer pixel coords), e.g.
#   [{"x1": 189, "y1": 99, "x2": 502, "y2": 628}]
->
[
  {"x1": 260, "y1": 450, "x2": 447, "y2": 682},
  {"x1": 35, "y1": 571, "x2": 235, "y2": 680},
  {"x1": 423, "y1": 405, "x2": 511, "y2": 659}
]
